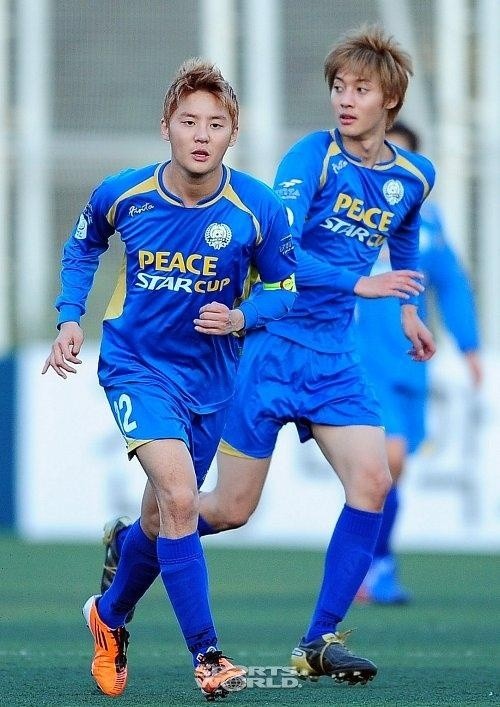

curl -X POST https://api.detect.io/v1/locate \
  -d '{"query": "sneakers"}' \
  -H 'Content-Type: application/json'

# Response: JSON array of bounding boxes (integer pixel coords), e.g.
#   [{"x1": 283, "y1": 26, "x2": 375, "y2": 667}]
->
[
  {"x1": 357, "y1": 557, "x2": 412, "y2": 607},
  {"x1": 193, "y1": 645, "x2": 247, "y2": 701},
  {"x1": 290, "y1": 632, "x2": 377, "y2": 687},
  {"x1": 100, "y1": 515, "x2": 137, "y2": 624},
  {"x1": 82, "y1": 593, "x2": 126, "y2": 695}
]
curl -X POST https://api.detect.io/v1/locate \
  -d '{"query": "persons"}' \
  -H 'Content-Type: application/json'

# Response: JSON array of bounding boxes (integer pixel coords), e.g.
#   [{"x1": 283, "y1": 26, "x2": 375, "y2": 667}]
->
[
  {"x1": 40, "y1": 57, "x2": 299, "y2": 702},
  {"x1": 98, "y1": 27, "x2": 441, "y2": 687},
  {"x1": 345, "y1": 119, "x2": 486, "y2": 608}
]
